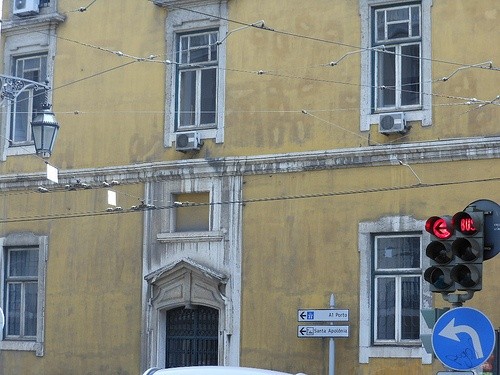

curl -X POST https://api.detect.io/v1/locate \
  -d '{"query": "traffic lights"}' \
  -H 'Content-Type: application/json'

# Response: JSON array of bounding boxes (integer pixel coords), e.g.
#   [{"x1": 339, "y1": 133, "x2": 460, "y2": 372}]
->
[
  {"x1": 450, "y1": 211, "x2": 483, "y2": 291},
  {"x1": 424, "y1": 215, "x2": 456, "y2": 292}
]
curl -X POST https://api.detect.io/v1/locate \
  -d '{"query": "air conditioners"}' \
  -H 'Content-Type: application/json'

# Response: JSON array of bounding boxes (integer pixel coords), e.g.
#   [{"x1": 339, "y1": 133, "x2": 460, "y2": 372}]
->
[
  {"x1": 12, "y1": 0, "x2": 40, "y2": 17},
  {"x1": 176, "y1": 131, "x2": 200, "y2": 153},
  {"x1": 378, "y1": 112, "x2": 407, "y2": 136}
]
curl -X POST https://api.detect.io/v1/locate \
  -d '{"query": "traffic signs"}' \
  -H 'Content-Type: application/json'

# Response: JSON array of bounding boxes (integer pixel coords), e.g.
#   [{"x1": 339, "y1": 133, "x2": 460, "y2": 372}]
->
[
  {"x1": 297, "y1": 309, "x2": 349, "y2": 322},
  {"x1": 297, "y1": 326, "x2": 349, "y2": 338}
]
